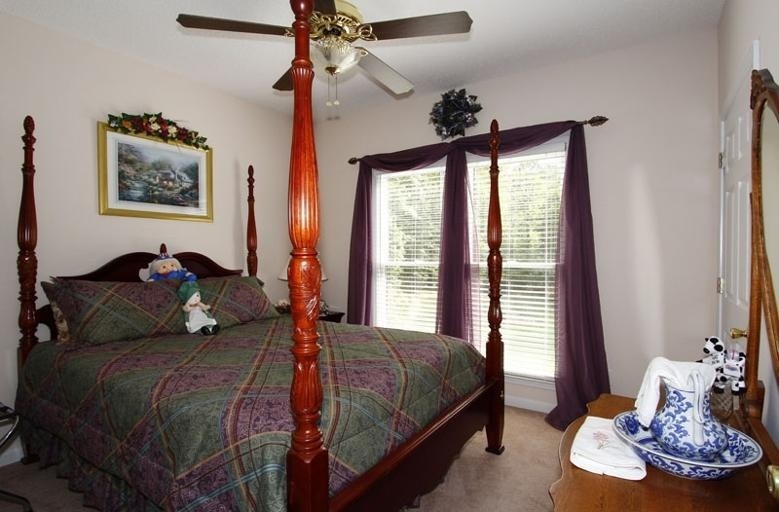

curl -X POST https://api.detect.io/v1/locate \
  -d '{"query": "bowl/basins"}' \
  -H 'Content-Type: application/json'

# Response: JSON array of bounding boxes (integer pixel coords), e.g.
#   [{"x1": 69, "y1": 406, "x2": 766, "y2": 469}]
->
[{"x1": 612, "y1": 408, "x2": 762, "y2": 480}]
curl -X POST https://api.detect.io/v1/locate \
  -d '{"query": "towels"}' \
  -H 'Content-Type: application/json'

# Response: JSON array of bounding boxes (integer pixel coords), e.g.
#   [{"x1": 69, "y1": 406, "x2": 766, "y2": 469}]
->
[
  {"x1": 571, "y1": 415, "x2": 648, "y2": 483},
  {"x1": 633, "y1": 351, "x2": 686, "y2": 428}
]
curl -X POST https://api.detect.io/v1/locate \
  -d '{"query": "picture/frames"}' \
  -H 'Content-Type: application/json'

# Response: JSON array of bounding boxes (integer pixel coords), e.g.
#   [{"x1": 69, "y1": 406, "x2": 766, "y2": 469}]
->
[{"x1": 96, "y1": 120, "x2": 214, "y2": 225}]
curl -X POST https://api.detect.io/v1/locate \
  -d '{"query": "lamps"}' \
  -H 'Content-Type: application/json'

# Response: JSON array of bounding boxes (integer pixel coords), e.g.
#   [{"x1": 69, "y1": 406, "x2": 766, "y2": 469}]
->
[
  {"x1": 276, "y1": 248, "x2": 329, "y2": 311},
  {"x1": 309, "y1": 41, "x2": 359, "y2": 78}
]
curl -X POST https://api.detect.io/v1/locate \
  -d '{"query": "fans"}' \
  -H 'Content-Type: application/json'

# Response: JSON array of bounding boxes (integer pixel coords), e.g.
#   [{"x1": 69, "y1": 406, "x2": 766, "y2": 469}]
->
[{"x1": 174, "y1": 0, "x2": 476, "y2": 97}]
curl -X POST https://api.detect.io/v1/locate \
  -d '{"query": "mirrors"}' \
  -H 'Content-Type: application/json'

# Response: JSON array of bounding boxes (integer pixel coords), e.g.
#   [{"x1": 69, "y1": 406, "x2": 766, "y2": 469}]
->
[{"x1": 733, "y1": 70, "x2": 778, "y2": 508}]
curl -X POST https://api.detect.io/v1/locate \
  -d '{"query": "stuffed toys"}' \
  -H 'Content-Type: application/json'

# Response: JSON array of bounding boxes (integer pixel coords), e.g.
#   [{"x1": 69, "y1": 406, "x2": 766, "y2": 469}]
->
[
  {"x1": 176, "y1": 281, "x2": 220, "y2": 336},
  {"x1": 139, "y1": 252, "x2": 198, "y2": 283}
]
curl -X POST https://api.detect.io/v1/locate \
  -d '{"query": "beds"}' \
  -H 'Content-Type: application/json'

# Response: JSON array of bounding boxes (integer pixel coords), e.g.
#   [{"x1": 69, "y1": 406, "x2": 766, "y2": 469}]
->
[{"x1": 14, "y1": 0, "x2": 508, "y2": 511}]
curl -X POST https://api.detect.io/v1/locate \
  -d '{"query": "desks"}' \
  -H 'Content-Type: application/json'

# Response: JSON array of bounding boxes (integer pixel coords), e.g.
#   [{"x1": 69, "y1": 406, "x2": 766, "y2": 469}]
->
[{"x1": 543, "y1": 390, "x2": 779, "y2": 512}]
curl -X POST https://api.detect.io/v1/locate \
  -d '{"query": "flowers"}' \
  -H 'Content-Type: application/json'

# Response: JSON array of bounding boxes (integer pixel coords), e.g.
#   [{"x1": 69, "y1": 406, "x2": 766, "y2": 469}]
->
[
  {"x1": 427, "y1": 87, "x2": 484, "y2": 143},
  {"x1": 107, "y1": 108, "x2": 214, "y2": 155}
]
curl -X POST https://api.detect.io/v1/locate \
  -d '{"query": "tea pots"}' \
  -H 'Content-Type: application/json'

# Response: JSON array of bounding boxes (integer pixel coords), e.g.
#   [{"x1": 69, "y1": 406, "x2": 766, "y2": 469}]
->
[{"x1": 650, "y1": 357, "x2": 729, "y2": 461}]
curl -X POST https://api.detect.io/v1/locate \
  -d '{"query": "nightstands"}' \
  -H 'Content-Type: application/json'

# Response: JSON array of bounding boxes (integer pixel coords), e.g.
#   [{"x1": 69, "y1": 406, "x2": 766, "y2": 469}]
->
[{"x1": 276, "y1": 305, "x2": 345, "y2": 324}]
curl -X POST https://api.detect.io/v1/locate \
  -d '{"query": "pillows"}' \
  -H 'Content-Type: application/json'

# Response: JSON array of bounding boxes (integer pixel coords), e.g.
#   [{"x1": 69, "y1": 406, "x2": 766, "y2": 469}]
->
[{"x1": 38, "y1": 270, "x2": 284, "y2": 351}]
